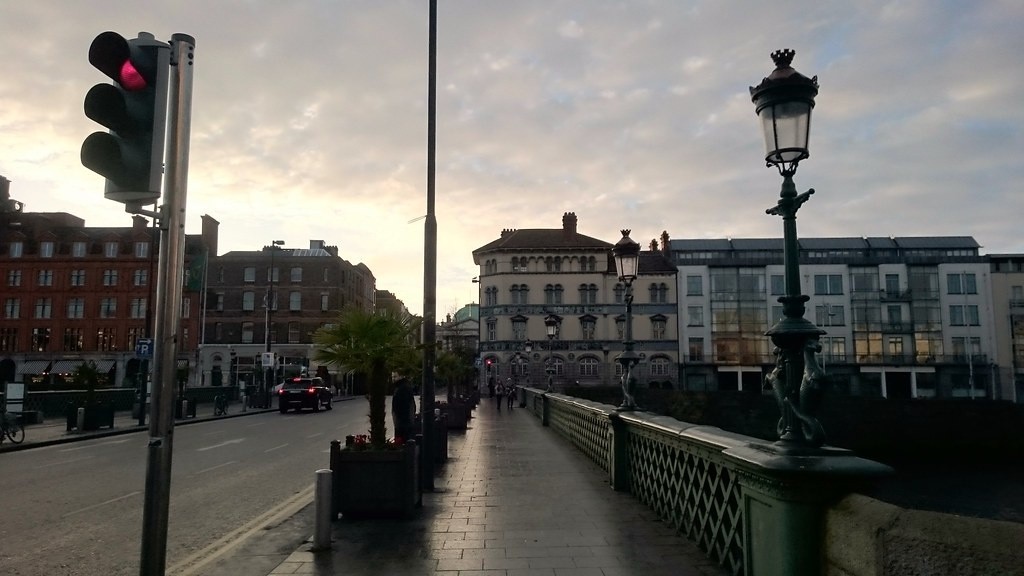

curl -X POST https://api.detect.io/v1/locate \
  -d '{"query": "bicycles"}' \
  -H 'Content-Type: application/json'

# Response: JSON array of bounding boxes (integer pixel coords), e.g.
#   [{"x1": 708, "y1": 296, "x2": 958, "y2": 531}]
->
[{"x1": 3, "y1": 411, "x2": 26, "y2": 444}]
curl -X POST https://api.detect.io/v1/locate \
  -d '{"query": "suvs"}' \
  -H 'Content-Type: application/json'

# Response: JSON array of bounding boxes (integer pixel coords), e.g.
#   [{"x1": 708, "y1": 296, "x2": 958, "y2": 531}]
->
[{"x1": 279, "y1": 376, "x2": 334, "y2": 414}]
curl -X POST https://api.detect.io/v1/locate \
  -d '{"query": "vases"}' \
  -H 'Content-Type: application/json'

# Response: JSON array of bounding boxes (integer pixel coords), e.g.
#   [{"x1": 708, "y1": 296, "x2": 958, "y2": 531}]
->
[{"x1": 339, "y1": 435, "x2": 422, "y2": 516}]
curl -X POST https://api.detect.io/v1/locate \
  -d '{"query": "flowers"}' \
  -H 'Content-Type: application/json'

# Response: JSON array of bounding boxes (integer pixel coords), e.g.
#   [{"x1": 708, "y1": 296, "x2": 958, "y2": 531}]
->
[{"x1": 353, "y1": 432, "x2": 401, "y2": 449}]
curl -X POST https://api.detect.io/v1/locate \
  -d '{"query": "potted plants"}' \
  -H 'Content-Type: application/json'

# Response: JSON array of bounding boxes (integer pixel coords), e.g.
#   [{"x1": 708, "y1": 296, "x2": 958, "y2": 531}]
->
[
  {"x1": 67, "y1": 363, "x2": 114, "y2": 429},
  {"x1": 415, "y1": 349, "x2": 480, "y2": 463}
]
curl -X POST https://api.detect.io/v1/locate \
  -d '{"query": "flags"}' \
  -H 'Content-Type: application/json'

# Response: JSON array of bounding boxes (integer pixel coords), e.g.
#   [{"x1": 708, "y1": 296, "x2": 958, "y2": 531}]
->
[{"x1": 188, "y1": 254, "x2": 204, "y2": 291}]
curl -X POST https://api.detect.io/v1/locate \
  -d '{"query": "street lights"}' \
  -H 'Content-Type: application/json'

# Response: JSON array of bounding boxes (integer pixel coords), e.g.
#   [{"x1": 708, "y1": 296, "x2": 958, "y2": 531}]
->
[
  {"x1": 542, "y1": 314, "x2": 557, "y2": 394},
  {"x1": 266, "y1": 239, "x2": 285, "y2": 353},
  {"x1": 515, "y1": 350, "x2": 522, "y2": 386},
  {"x1": 746, "y1": 46, "x2": 826, "y2": 457},
  {"x1": 609, "y1": 227, "x2": 644, "y2": 411},
  {"x1": 523, "y1": 338, "x2": 532, "y2": 387}
]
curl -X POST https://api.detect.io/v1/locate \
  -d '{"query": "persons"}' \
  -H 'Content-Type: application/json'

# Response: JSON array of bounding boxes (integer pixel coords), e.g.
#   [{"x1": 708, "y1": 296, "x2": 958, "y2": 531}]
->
[
  {"x1": 488, "y1": 376, "x2": 515, "y2": 411},
  {"x1": 391, "y1": 369, "x2": 416, "y2": 444}
]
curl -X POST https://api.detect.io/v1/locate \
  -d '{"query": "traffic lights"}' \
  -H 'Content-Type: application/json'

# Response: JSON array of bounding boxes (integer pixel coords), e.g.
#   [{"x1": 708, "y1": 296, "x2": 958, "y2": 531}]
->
[{"x1": 80, "y1": 26, "x2": 173, "y2": 216}]
